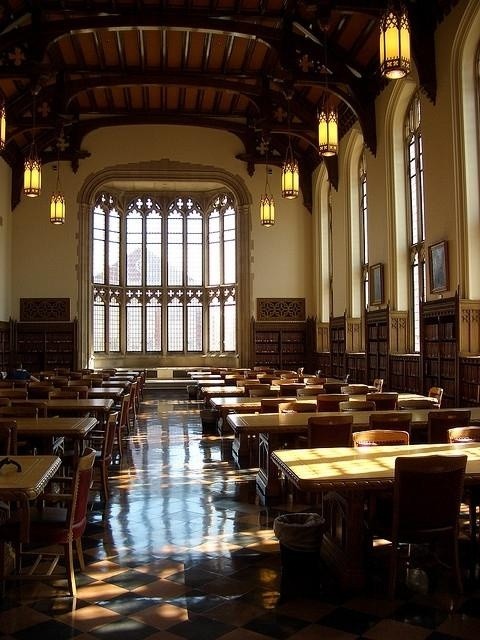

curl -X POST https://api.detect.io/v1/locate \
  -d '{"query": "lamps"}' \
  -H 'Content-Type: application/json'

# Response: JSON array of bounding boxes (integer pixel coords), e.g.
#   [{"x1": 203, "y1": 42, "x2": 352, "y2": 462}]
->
[
  {"x1": 380, "y1": 1, "x2": 411, "y2": 80},
  {"x1": 259, "y1": 136, "x2": 275, "y2": 227},
  {"x1": 49, "y1": 140, "x2": 65, "y2": 224},
  {"x1": 23, "y1": 84, "x2": 41, "y2": 198},
  {"x1": 0, "y1": 105, "x2": 8, "y2": 150},
  {"x1": 281, "y1": 86, "x2": 300, "y2": 200},
  {"x1": 317, "y1": 90, "x2": 337, "y2": 158}
]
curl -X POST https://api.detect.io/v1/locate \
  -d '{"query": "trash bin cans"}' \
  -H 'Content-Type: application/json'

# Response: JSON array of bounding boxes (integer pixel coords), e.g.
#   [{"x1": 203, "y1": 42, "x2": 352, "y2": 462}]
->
[
  {"x1": 272, "y1": 513, "x2": 327, "y2": 570},
  {"x1": 200, "y1": 409, "x2": 220, "y2": 434},
  {"x1": 187, "y1": 384, "x2": 198, "y2": 400}
]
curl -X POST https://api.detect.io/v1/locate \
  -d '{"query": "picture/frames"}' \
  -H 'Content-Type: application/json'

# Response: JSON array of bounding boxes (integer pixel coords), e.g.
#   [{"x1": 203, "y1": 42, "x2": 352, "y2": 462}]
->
[
  {"x1": 369, "y1": 264, "x2": 384, "y2": 306},
  {"x1": 428, "y1": 241, "x2": 450, "y2": 292}
]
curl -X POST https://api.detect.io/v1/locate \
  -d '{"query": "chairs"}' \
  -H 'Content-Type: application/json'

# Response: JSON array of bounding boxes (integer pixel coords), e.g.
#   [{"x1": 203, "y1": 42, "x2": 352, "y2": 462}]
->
[{"x1": 0, "y1": 370, "x2": 148, "y2": 595}]
